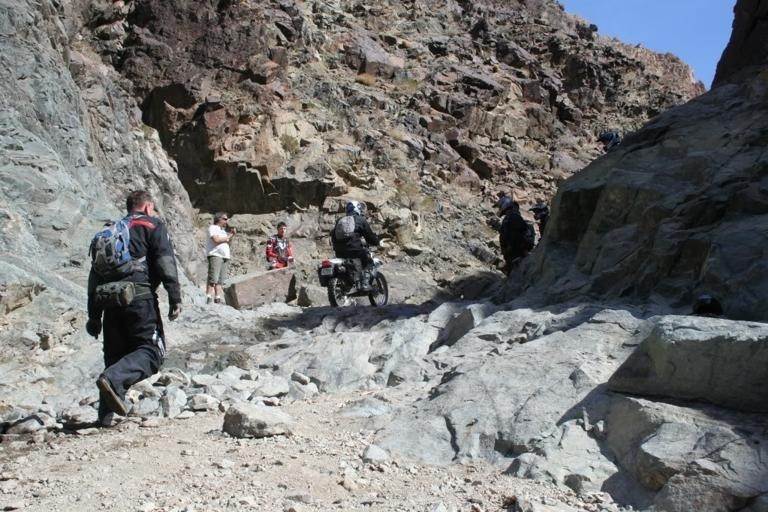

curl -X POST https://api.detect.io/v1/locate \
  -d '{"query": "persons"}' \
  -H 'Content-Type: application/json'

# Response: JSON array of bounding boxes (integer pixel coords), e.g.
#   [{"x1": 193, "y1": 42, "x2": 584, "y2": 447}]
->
[
  {"x1": 266, "y1": 222, "x2": 294, "y2": 268},
  {"x1": 88, "y1": 190, "x2": 182, "y2": 425},
  {"x1": 528, "y1": 203, "x2": 549, "y2": 238},
  {"x1": 488, "y1": 198, "x2": 528, "y2": 268},
  {"x1": 206, "y1": 212, "x2": 237, "y2": 304},
  {"x1": 330, "y1": 200, "x2": 384, "y2": 292}
]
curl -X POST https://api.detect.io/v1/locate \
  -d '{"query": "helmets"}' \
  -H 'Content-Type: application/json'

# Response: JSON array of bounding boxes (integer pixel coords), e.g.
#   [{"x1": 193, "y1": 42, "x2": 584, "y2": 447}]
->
[
  {"x1": 492, "y1": 198, "x2": 512, "y2": 216},
  {"x1": 529, "y1": 204, "x2": 548, "y2": 220},
  {"x1": 693, "y1": 294, "x2": 722, "y2": 315},
  {"x1": 346, "y1": 201, "x2": 361, "y2": 215}
]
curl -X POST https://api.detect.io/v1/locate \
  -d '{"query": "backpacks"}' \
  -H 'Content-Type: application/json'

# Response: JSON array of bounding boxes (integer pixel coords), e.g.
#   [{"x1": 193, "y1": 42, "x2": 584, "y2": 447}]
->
[{"x1": 89, "y1": 215, "x2": 148, "y2": 308}]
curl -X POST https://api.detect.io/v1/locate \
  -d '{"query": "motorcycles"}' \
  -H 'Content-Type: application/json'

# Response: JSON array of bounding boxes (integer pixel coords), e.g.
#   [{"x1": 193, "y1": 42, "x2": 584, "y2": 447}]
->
[{"x1": 317, "y1": 244, "x2": 389, "y2": 308}]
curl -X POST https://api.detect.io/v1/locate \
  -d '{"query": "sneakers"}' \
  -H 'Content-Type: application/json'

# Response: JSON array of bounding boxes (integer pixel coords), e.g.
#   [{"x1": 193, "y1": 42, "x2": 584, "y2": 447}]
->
[
  {"x1": 97, "y1": 375, "x2": 127, "y2": 416},
  {"x1": 208, "y1": 297, "x2": 220, "y2": 305},
  {"x1": 361, "y1": 285, "x2": 374, "y2": 292}
]
